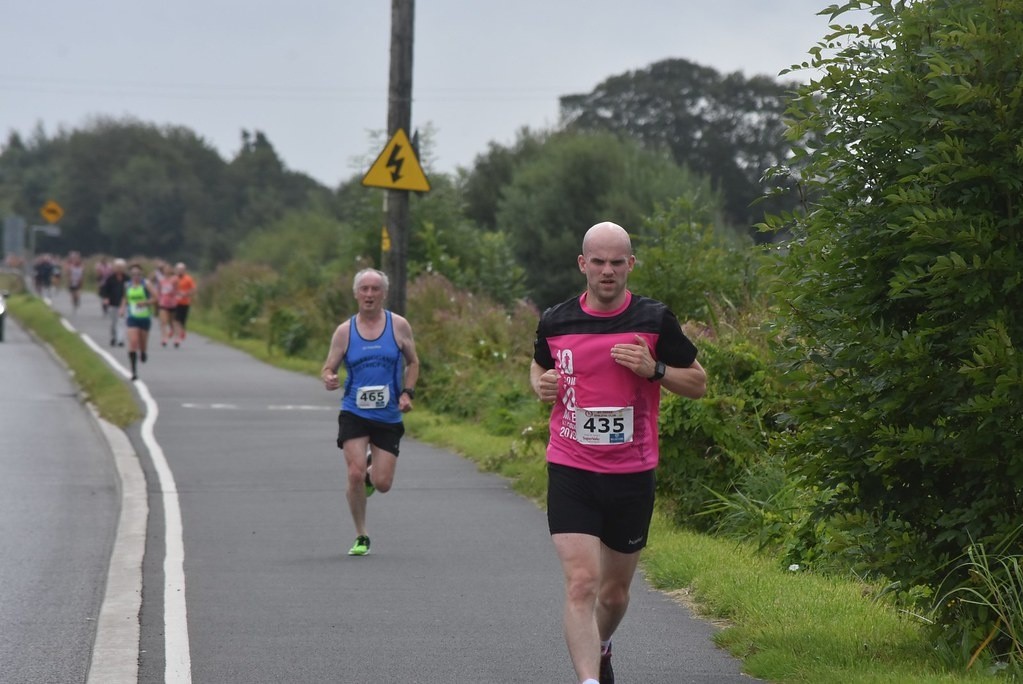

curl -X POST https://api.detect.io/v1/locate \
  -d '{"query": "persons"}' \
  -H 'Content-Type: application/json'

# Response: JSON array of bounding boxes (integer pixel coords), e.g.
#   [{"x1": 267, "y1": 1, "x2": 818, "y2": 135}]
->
[
  {"x1": 66, "y1": 251, "x2": 84, "y2": 309},
  {"x1": 33, "y1": 253, "x2": 63, "y2": 300},
  {"x1": 118, "y1": 263, "x2": 158, "y2": 381},
  {"x1": 530, "y1": 222, "x2": 707, "y2": 684},
  {"x1": 95, "y1": 257, "x2": 112, "y2": 286},
  {"x1": 320, "y1": 267, "x2": 419, "y2": 556},
  {"x1": 100, "y1": 258, "x2": 130, "y2": 347},
  {"x1": 149, "y1": 259, "x2": 195, "y2": 348}
]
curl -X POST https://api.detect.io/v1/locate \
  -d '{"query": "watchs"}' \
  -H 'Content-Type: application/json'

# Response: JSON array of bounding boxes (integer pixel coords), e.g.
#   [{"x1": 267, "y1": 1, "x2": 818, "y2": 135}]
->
[
  {"x1": 648, "y1": 361, "x2": 666, "y2": 383},
  {"x1": 403, "y1": 388, "x2": 415, "y2": 399}
]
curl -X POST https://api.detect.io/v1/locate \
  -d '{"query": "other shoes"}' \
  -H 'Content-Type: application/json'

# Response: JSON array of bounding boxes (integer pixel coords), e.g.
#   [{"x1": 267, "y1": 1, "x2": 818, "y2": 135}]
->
[{"x1": 110, "y1": 329, "x2": 186, "y2": 382}]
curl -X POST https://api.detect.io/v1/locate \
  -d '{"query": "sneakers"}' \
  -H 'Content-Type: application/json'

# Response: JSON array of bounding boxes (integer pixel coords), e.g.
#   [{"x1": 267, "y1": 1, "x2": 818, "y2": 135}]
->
[
  {"x1": 366, "y1": 451, "x2": 375, "y2": 498},
  {"x1": 348, "y1": 535, "x2": 372, "y2": 555},
  {"x1": 598, "y1": 641, "x2": 614, "y2": 684}
]
